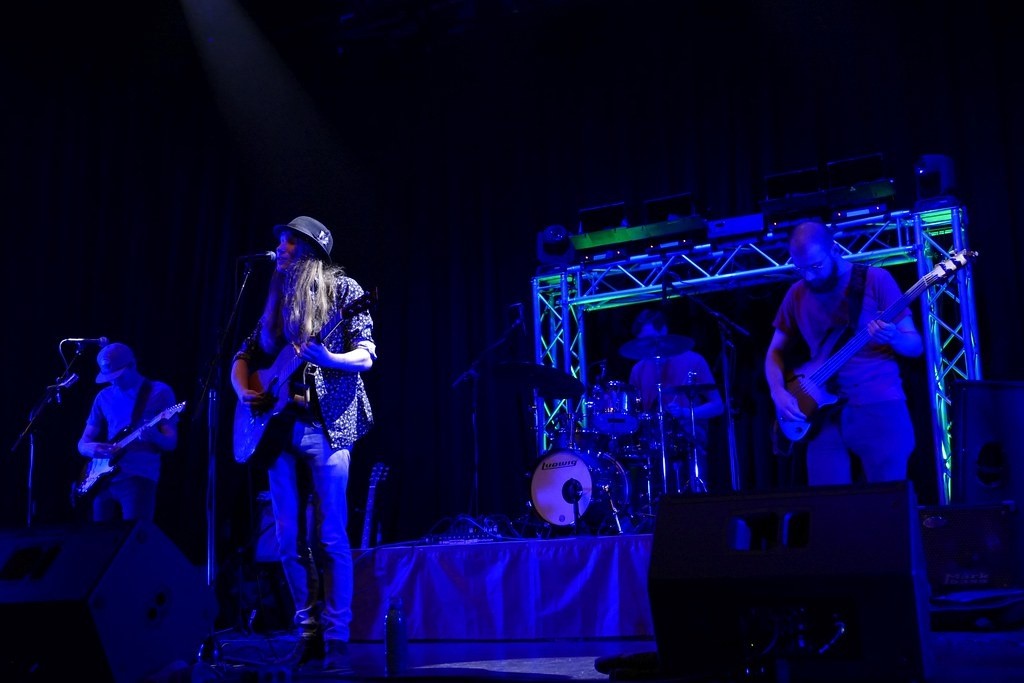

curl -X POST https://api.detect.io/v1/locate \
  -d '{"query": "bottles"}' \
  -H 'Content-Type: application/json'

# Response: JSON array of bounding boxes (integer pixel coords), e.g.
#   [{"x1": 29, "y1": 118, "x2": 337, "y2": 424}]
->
[{"x1": 386, "y1": 595, "x2": 408, "y2": 673}]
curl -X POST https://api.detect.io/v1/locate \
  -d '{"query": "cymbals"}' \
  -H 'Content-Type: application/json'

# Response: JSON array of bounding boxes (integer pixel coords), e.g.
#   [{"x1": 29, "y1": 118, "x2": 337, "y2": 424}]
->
[
  {"x1": 662, "y1": 384, "x2": 718, "y2": 397},
  {"x1": 497, "y1": 362, "x2": 585, "y2": 400},
  {"x1": 619, "y1": 335, "x2": 696, "y2": 361}
]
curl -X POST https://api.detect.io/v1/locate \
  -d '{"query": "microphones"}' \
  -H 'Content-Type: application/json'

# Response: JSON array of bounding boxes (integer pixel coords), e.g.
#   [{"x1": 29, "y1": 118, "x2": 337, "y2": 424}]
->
[
  {"x1": 67, "y1": 337, "x2": 111, "y2": 348},
  {"x1": 240, "y1": 250, "x2": 276, "y2": 264}
]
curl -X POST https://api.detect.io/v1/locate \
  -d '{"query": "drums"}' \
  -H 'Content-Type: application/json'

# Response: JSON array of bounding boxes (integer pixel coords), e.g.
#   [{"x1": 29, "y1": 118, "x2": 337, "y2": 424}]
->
[
  {"x1": 530, "y1": 449, "x2": 630, "y2": 530},
  {"x1": 588, "y1": 380, "x2": 643, "y2": 441},
  {"x1": 618, "y1": 444, "x2": 663, "y2": 491},
  {"x1": 551, "y1": 413, "x2": 600, "y2": 444}
]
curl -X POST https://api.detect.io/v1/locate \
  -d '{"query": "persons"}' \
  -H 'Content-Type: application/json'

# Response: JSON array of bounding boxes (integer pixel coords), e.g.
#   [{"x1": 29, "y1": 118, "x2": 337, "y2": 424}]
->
[
  {"x1": 77, "y1": 343, "x2": 181, "y2": 524},
  {"x1": 620, "y1": 321, "x2": 725, "y2": 493},
  {"x1": 230, "y1": 217, "x2": 377, "y2": 675},
  {"x1": 765, "y1": 222, "x2": 925, "y2": 487}
]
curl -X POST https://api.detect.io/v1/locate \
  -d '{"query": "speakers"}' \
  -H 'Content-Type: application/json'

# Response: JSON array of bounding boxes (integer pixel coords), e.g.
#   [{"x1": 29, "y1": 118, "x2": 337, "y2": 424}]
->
[
  {"x1": 0, "y1": 518, "x2": 220, "y2": 683},
  {"x1": 952, "y1": 379, "x2": 1024, "y2": 589},
  {"x1": 649, "y1": 478, "x2": 935, "y2": 683},
  {"x1": 917, "y1": 501, "x2": 1017, "y2": 596}
]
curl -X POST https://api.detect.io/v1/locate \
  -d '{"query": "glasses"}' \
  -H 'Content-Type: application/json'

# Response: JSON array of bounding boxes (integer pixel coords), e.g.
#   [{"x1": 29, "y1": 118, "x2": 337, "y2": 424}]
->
[{"x1": 791, "y1": 252, "x2": 831, "y2": 272}]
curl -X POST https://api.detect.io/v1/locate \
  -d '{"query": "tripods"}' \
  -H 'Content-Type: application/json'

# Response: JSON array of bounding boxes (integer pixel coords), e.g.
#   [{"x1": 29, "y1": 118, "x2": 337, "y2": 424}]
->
[{"x1": 678, "y1": 371, "x2": 710, "y2": 495}]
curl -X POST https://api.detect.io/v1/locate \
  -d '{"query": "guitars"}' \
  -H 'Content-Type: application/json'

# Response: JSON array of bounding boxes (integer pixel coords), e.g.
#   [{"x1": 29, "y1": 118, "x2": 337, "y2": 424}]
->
[
  {"x1": 775, "y1": 249, "x2": 967, "y2": 443},
  {"x1": 76, "y1": 399, "x2": 187, "y2": 498},
  {"x1": 232, "y1": 290, "x2": 380, "y2": 465}
]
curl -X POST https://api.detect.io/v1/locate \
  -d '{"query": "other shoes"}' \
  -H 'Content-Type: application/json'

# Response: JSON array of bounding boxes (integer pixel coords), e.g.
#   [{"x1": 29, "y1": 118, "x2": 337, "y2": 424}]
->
[
  {"x1": 323, "y1": 640, "x2": 352, "y2": 672},
  {"x1": 274, "y1": 637, "x2": 324, "y2": 672}
]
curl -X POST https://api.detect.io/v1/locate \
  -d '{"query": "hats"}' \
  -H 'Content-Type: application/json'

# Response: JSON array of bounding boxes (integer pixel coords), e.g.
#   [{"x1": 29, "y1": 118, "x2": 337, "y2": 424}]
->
[
  {"x1": 273, "y1": 216, "x2": 333, "y2": 264},
  {"x1": 96, "y1": 343, "x2": 135, "y2": 383}
]
